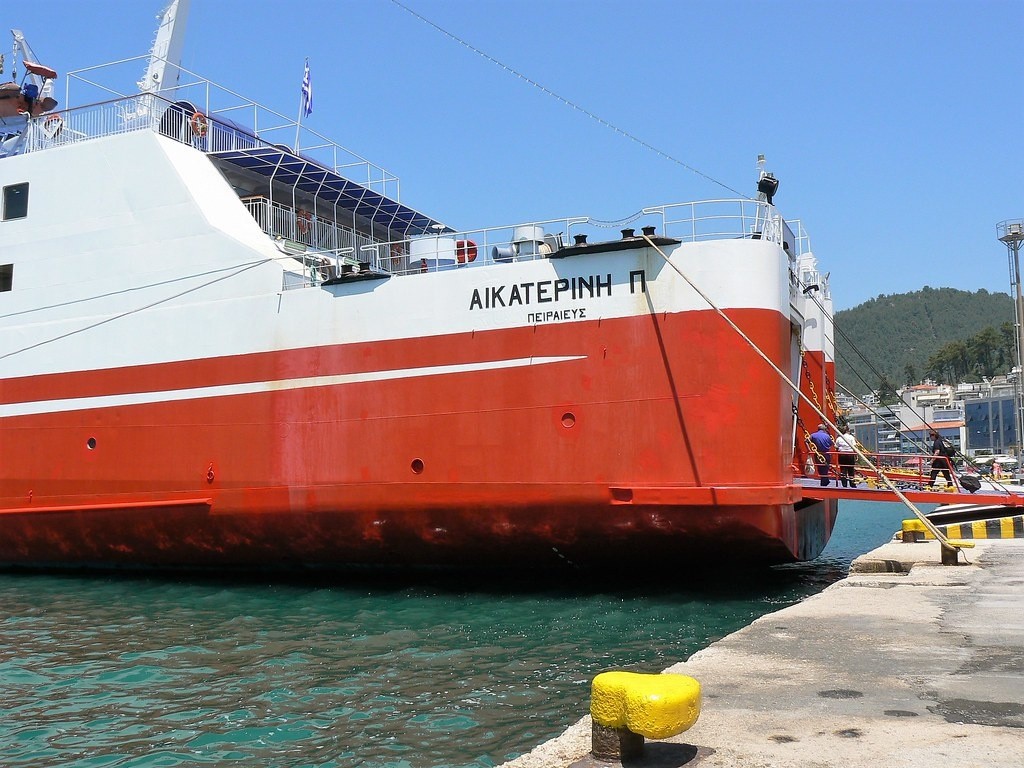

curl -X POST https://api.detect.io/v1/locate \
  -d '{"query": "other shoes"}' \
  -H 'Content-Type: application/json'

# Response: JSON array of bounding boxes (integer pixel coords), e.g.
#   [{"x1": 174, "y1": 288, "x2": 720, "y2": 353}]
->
[
  {"x1": 944, "y1": 485, "x2": 955, "y2": 493},
  {"x1": 922, "y1": 485, "x2": 934, "y2": 492}
]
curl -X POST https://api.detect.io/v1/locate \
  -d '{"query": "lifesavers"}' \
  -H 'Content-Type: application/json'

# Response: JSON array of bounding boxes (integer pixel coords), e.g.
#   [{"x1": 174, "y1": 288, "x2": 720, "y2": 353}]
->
[
  {"x1": 297, "y1": 209, "x2": 312, "y2": 234},
  {"x1": 391, "y1": 244, "x2": 402, "y2": 265},
  {"x1": 43, "y1": 114, "x2": 64, "y2": 138},
  {"x1": 191, "y1": 112, "x2": 207, "y2": 137}
]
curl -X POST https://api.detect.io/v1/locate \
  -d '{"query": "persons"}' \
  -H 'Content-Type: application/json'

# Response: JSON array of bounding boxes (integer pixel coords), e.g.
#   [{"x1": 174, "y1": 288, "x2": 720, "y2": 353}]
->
[
  {"x1": 833, "y1": 425, "x2": 858, "y2": 487},
  {"x1": 805, "y1": 423, "x2": 832, "y2": 486},
  {"x1": 928, "y1": 430, "x2": 953, "y2": 491}
]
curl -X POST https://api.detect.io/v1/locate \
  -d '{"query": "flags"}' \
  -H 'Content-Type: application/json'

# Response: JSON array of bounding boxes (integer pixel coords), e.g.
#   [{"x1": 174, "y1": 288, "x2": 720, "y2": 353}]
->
[{"x1": 302, "y1": 57, "x2": 312, "y2": 118}]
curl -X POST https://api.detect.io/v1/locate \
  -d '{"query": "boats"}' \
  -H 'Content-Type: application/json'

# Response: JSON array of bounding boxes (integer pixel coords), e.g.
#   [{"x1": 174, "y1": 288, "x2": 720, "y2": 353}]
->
[{"x1": 0, "y1": 0, "x2": 843, "y2": 567}]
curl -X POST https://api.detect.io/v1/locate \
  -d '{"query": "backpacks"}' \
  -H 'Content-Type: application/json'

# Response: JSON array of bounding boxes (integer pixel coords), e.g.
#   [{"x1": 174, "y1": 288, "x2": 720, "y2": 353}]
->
[{"x1": 939, "y1": 438, "x2": 956, "y2": 456}]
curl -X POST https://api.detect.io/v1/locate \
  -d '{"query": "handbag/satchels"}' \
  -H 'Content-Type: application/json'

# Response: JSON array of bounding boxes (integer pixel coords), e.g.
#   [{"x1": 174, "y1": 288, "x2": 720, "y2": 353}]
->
[{"x1": 805, "y1": 454, "x2": 815, "y2": 475}]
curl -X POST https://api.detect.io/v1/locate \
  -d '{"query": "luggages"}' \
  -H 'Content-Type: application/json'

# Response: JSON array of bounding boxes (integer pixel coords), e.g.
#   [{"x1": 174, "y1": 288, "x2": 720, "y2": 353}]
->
[{"x1": 953, "y1": 473, "x2": 981, "y2": 495}]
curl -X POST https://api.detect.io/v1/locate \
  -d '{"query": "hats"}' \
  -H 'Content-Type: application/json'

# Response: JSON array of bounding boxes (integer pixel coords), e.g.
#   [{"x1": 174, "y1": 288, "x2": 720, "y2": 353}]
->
[
  {"x1": 818, "y1": 424, "x2": 827, "y2": 429},
  {"x1": 929, "y1": 430, "x2": 939, "y2": 435}
]
